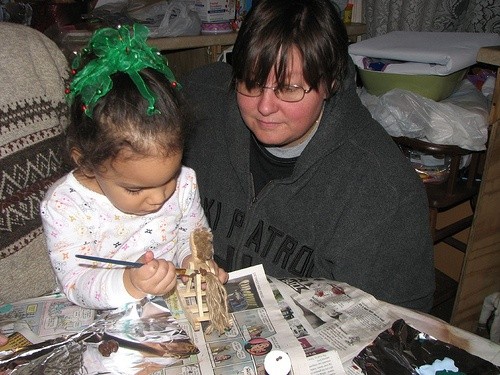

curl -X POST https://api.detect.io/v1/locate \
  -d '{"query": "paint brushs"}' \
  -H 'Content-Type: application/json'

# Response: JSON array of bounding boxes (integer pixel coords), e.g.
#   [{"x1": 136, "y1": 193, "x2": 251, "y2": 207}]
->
[{"x1": 75, "y1": 254, "x2": 194, "y2": 275}]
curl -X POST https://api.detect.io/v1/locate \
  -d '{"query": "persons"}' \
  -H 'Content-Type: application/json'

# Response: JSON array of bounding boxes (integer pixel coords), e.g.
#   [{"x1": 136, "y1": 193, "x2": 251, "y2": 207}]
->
[
  {"x1": 41, "y1": 22, "x2": 229, "y2": 311},
  {"x1": 182, "y1": 0, "x2": 438, "y2": 318}
]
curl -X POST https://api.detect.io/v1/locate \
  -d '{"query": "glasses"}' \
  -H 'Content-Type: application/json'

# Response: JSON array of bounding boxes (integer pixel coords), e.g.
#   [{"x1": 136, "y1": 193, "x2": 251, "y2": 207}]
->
[{"x1": 235, "y1": 72, "x2": 326, "y2": 103}]
